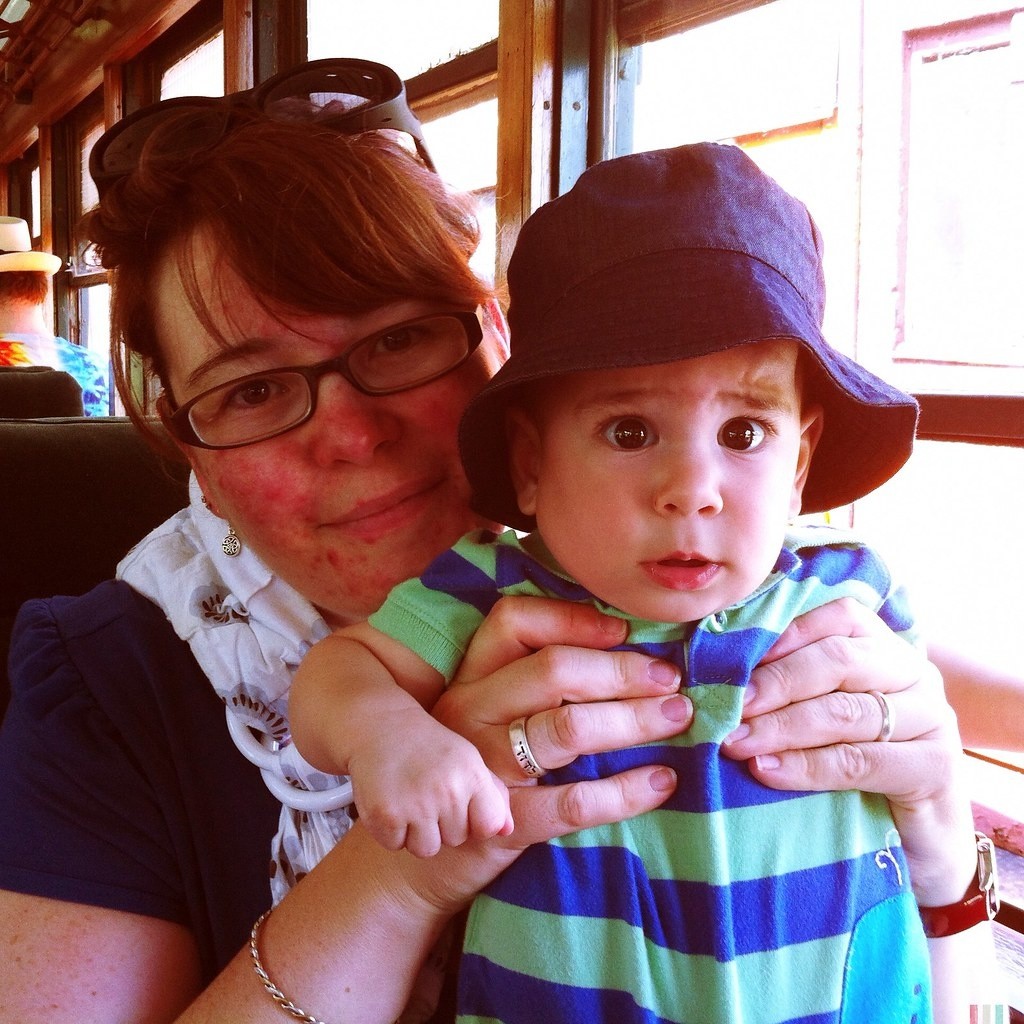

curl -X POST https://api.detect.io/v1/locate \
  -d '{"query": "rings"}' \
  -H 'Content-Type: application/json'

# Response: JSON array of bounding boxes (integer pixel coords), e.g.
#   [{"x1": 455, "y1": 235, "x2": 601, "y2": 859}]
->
[
  {"x1": 507, "y1": 718, "x2": 547, "y2": 780},
  {"x1": 865, "y1": 689, "x2": 890, "y2": 744}
]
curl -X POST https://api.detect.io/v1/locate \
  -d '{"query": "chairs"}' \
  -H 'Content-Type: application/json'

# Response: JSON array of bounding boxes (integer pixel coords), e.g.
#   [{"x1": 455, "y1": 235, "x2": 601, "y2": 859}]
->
[
  {"x1": 0, "y1": 416, "x2": 194, "y2": 713},
  {"x1": 0, "y1": 366, "x2": 85, "y2": 416}
]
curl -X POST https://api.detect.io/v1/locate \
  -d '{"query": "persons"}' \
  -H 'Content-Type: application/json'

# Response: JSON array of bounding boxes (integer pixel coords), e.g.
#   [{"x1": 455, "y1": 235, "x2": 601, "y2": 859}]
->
[
  {"x1": 0, "y1": 58, "x2": 1009, "y2": 1024},
  {"x1": 0, "y1": 215, "x2": 111, "y2": 418},
  {"x1": 285, "y1": 144, "x2": 932, "y2": 1021}
]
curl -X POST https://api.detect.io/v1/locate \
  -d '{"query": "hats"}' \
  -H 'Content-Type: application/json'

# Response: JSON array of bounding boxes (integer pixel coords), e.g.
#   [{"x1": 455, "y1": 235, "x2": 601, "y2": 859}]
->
[
  {"x1": 456, "y1": 141, "x2": 920, "y2": 532},
  {"x1": 0, "y1": 214, "x2": 62, "y2": 277}
]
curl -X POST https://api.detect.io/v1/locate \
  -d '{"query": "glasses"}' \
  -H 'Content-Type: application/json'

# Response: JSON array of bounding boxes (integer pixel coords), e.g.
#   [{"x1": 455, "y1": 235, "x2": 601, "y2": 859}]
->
[
  {"x1": 166, "y1": 307, "x2": 483, "y2": 450},
  {"x1": 84, "y1": 56, "x2": 448, "y2": 283}
]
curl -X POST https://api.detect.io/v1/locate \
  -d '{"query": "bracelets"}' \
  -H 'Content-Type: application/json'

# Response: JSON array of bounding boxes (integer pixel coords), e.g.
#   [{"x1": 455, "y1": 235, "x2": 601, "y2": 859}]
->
[{"x1": 248, "y1": 903, "x2": 328, "y2": 1024}]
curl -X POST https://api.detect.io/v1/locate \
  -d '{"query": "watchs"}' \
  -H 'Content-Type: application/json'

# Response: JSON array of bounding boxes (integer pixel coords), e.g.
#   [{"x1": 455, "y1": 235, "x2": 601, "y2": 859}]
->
[{"x1": 917, "y1": 830, "x2": 1001, "y2": 938}]
